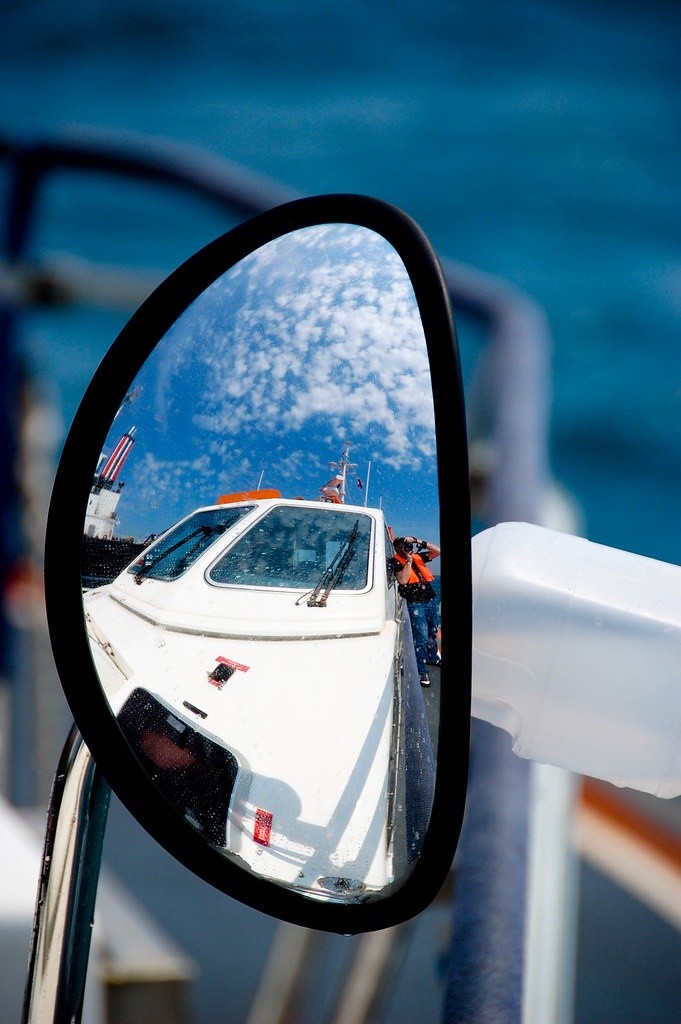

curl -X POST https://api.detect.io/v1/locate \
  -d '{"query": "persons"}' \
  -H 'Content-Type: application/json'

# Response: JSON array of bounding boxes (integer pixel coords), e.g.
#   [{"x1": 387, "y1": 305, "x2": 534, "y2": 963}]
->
[{"x1": 391, "y1": 537, "x2": 440, "y2": 686}]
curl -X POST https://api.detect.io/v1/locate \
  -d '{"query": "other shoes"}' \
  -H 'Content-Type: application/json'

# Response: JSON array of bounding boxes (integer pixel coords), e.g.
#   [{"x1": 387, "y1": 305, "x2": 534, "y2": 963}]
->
[{"x1": 421, "y1": 672, "x2": 433, "y2": 688}]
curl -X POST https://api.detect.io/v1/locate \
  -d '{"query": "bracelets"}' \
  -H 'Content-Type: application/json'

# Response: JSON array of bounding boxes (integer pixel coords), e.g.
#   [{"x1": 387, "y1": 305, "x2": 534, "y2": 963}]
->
[{"x1": 421, "y1": 541, "x2": 427, "y2": 548}]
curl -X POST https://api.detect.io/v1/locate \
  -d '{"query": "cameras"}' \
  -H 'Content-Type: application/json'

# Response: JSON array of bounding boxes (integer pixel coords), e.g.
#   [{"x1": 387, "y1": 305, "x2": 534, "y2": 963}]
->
[{"x1": 403, "y1": 543, "x2": 414, "y2": 552}]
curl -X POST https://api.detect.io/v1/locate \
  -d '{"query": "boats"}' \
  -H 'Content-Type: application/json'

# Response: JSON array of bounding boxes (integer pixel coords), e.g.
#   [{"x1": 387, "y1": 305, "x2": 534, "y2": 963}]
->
[{"x1": 79, "y1": 440, "x2": 428, "y2": 904}]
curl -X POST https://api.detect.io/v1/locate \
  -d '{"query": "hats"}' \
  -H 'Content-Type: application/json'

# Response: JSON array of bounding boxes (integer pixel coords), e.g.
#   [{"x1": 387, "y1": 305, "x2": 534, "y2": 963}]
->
[{"x1": 393, "y1": 537, "x2": 405, "y2": 546}]
